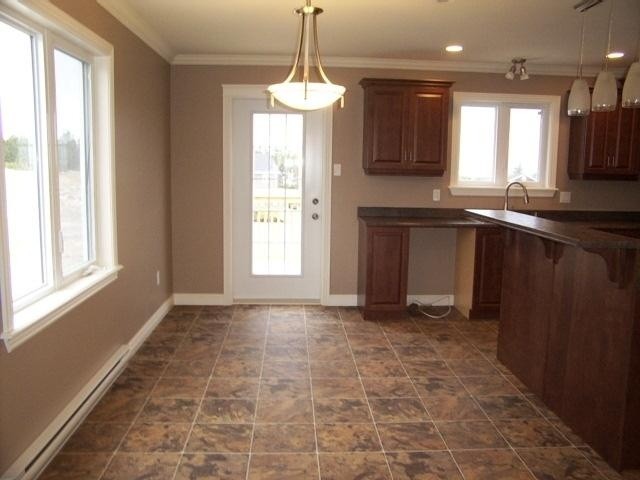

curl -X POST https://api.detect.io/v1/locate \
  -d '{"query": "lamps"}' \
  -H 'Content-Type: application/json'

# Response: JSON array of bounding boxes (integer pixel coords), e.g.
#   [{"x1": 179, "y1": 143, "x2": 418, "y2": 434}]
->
[
  {"x1": 264, "y1": 2, "x2": 348, "y2": 111},
  {"x1": 504, "y1": 57, "x2": 532, "y2": 83},
  {"x1": 566, "y1": 1, "x2": 639, "y2": 116}
]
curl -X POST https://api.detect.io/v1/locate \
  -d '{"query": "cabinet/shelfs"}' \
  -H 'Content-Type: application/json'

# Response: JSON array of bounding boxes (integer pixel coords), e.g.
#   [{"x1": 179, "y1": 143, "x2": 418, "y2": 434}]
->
[
  {"x1": 358, "y1": 215, "x2": 410, "y2": 317},
  {"x1": 454, "y1": 224, "x2": 507, "y2": 320},
  {"x1": 358, "y1": 75, "x2": 455, "y2": 178},
  {"x1": 568, "y1": 87, "x2": 640, "y2": 180}
]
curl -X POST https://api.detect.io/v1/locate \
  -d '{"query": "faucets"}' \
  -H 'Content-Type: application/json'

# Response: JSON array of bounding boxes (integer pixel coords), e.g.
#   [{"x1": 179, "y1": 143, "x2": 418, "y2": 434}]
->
[{"x1": 504, "y1": 181, "x2": 529, "y2": 211}]
